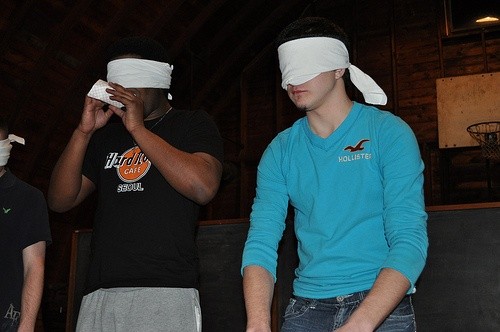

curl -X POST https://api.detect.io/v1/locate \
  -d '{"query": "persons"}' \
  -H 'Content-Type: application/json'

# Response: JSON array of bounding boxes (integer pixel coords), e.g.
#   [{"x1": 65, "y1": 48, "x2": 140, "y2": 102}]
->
[
  {"x1": 47, "y1": 36, "x2": 227, "y2": 332},
  {"x1": 241, "y1": 16, "x2": 429, "y2": 332},
  {"x1": 0, "y1": 119, "x2": 54, "y2": 332}
]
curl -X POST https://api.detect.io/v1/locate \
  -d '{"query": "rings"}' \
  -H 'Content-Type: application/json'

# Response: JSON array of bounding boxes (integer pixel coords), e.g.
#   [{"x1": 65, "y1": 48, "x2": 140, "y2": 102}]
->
[{"x1": 134, "y1": 94, "x2": 136, "y2": 97}]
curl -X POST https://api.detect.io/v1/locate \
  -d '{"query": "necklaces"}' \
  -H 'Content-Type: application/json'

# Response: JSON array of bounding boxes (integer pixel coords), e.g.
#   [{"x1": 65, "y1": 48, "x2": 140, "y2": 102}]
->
[{"x1": 133, "y1": 107, "x2": 172, "y2": 146}]
